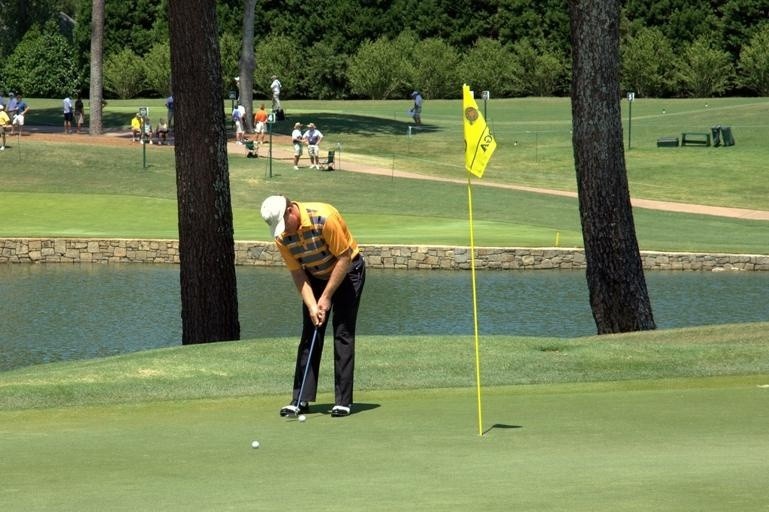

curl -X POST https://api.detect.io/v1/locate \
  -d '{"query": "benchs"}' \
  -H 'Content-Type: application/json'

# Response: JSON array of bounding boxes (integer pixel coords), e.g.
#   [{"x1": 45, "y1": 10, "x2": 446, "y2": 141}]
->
[{"x1": 657, "y1": 137, "x2": 679, "y2": 147}]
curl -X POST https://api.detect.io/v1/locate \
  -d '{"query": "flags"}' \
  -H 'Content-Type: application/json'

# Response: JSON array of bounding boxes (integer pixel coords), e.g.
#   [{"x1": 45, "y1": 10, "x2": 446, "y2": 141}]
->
[{"x1": 463, "y1": 83, "x2": 497, "y2": 179}]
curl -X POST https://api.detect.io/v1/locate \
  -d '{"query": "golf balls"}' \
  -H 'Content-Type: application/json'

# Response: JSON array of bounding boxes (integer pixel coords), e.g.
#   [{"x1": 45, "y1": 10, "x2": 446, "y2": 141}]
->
[
  {"x1": 252, "y1": 440, "x2": 258, "y2": 449},
  {"x1": 298, "y1": 414, "x2": 305, "y2": 421}
]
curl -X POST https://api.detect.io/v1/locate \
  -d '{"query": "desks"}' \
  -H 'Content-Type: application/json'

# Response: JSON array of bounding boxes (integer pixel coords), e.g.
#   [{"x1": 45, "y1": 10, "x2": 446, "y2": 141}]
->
[{"x1": 681, "y1": 131, "x2": 711, "y2": 147}]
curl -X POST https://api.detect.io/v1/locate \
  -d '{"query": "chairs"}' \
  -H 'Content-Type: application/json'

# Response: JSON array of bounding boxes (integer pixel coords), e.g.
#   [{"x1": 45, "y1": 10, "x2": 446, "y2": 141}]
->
[
  {"x1": 244, "y1": 141, "x2": 260, "y2": 159},
  {"x1": 320, "y1": 151, "x2": 336, "y2": 171}
]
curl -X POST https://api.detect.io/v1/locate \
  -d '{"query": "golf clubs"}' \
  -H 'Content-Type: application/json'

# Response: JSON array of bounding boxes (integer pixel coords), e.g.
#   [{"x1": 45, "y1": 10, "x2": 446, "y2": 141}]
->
[{"x1": 286, "y1": 324, "x2": 317, "y2": 418}]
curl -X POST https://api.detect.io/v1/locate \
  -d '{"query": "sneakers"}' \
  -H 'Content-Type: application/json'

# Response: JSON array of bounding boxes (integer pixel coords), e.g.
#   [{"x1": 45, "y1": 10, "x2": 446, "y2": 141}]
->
[
  {"x1": 331, "y1": 403, "x2": 352, "y2": 416},
  {"x1": 280, "y1": 400, "x2": 309, "y2": 416}
]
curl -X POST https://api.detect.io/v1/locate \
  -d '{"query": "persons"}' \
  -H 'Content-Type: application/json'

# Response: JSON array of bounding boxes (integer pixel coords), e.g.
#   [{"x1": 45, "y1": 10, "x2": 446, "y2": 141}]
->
[
  {"x1": 410, "y1": 92, "x2": 423, "y2": 126},
  {"x1": 101, "y1": 98, "x2": 107, "y2": 110},
  {"x1": 0, "y1": 92, "x2": 30, "y2": 151},
  {"x1": 231, "y1": 96, "x2": 269, "y2": 146},
  {"x1": 302, "y1": 121, "x2": 323, "y2": 170},
  {"x1": 234, "y1": 76, "x2": 240, "y2": 104},
  {"x1": 131, "y1": 112, "x2": 168, "y2": 145},
  {"x1": 75, "y1": 95, "x2": 84, "y2": 134},
  {"x1": 260, "y1": 195, "x2": 365, "y2": 418},
  {"x1": 63, "y1": 94, "x2": 74, "y2": 134},
  {"x1": 270, "y1": 74, "x2": 283, "y2": 112},
  {"x1": 292, "y1": 122, "x2": 306, "y2": 170}
]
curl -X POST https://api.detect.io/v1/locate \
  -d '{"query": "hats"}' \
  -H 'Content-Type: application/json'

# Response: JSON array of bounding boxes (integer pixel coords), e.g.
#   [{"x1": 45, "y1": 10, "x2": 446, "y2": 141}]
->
[
  {"x1": 294, "y1": 122, "x2": 303, "y2": 128},
  {"x1": 412, "y1": 91, "x2": 418, "y2": 96},
  {"x1": 306, "y1": 123, "x2": 316, "y2": 128},
  {"x1": 260, "y1": 195, "x2": 287, "y2": 237}
]
